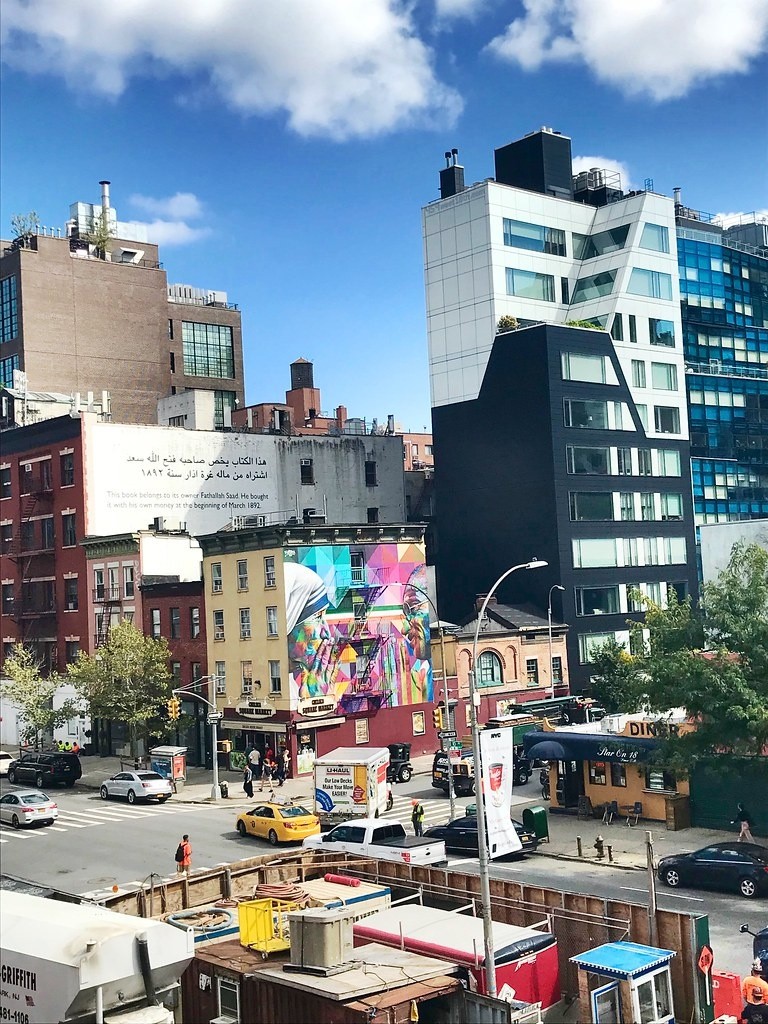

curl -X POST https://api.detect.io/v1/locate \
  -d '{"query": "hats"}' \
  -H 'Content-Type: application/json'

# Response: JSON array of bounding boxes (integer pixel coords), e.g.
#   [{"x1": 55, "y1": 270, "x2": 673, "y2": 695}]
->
[{"x1": 751, "y1": 966, "x2": 762, "y2": 971}]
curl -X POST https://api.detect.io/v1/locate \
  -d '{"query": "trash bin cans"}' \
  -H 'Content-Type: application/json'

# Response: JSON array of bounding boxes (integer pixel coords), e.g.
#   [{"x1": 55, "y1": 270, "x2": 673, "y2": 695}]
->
[
  {"x1": 220, "y1": 780, "x2": 228, "y2": 798},
  {"x1": 388, "y1": 743, "x2": 412, "y2": 762}
]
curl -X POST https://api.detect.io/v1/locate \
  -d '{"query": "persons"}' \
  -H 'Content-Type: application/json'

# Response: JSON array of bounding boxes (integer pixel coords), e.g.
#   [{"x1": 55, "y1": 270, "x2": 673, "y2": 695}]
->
[
  {"x1": 259, "y1": 759, "x2": 273, "y2": 792},
  {"x1": 731, "y1": 803, "x2": 756, "y2": 844},
  {"x1": 735, "y1": 962, "x2": 768, "y2": 1024},
  {"x1": 249, "y1": 745, "x2": 260, "y2": 780},
  {"x1": 243, "y1": 765, "x2": 254, "y2": 799},
  {"x1": 411, "y1": 799, "x2": 425, "y2": 837},
  {"x1": 50, "y1": 739, "x2": 79, "y2": 754},
  {"x1": 265, "y1": 747, "x2": 273, "y2": 759},
  {"x1": 276, "y1": 751, "x2": 284, "y2": 787},
  {"x1": 283, "y1": 750, "x2": 291, "y2": 779},
  {"x1": 244, "y1": 742, "x2": 253, "y2": 763},
  {"x1": 177, "y1": 835, "x2": 192, "y2": 875}
]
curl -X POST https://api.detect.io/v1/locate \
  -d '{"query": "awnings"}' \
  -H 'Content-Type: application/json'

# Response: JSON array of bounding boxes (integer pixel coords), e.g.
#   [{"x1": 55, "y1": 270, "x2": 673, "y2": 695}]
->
[{"x1": 523, "y1": 731, "x2": 681, "y2": 767}]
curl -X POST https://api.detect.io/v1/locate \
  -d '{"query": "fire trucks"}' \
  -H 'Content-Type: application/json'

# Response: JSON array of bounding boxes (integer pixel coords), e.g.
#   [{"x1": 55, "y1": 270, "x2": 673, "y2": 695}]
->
[{"x1": 431, "y1": 747, "x2": 533, "y2": 797}]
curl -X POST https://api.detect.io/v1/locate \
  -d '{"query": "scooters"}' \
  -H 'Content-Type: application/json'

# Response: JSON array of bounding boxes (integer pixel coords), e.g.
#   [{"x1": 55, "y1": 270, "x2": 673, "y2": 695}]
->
[
  {"x1": 738, "y1": 921, "x2": 768, "y2": 984},
  {"x1": 539, "y1": 768, "x2": 550, "y2": 801}
]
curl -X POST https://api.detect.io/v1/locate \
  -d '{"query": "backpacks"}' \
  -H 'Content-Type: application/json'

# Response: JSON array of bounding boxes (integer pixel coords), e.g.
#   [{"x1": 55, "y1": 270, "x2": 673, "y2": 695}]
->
[{"x1": 175, "y1": 843, "x2": 188, "y2": 862}]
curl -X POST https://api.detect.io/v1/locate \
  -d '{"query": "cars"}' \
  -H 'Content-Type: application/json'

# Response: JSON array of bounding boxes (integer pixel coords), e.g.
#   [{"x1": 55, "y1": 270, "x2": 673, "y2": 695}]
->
[
  {"x1": 0, "y1": 750, "x2": 16, "y2": 777},
  {"x1": 236, "y1": 802, "x2": 321, "y2": 846},
  {"x1": 99, "y1": 770, "x2": 173, "y2": 805},
  {"x1": 657, "y1": 841, "x2": 768, "y2": 900},
  {"x1": 1, "y1": 790, "x2": 59, "y2": 829},
  {"x1": 422, "y1": 813, "x2": 538, "y2": 862}
]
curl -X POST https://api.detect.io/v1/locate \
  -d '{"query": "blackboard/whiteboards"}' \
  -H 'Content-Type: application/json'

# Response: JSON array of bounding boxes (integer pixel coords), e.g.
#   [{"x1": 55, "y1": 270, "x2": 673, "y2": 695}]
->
[{"x1": 578, "y1": 795, "x2": 594, "y2": 818}]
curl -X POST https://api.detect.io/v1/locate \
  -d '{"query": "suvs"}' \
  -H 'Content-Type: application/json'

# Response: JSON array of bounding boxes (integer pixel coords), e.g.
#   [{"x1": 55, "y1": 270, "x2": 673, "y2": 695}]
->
[{"x1": 7, "y1": 751, "x2": 83, "y2": 790}]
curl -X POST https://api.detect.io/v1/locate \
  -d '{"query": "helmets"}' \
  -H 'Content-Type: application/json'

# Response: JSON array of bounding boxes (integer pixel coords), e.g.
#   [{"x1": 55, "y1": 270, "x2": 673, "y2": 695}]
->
[
  {"x1": 412, "y1": 799, "x2": 418, "y2": 807},
  {"x1": 751, "y1": 987, "x2": 764, "y2": 996}
]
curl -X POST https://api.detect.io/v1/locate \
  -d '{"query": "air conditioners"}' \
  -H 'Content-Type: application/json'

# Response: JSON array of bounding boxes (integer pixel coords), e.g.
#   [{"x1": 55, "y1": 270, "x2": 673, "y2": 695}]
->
[
  {"x1": 301, "y1": 460, "x2": 310, "y2": 466},
  {"x1": 241, "y1": 631, "x2": 250, "y2": 637},
  {"x1": 244, "y1": 685, "x2": 252, "y2": 692},
  {"x1": 25, "y1": 464, "x2": 33, "y2": 472},
  {"x1": 213, "y1": 585, "x2": 222, "y2": 592},
  {"x1": 217, "y1": 685, "x2": 226, "y2": 693},
  {"x1": 215, "y1": 631, "x2": 224, "y2": 639},
  {"x1": 266, "y1": 580, "x2": 276, "y2": 587}
]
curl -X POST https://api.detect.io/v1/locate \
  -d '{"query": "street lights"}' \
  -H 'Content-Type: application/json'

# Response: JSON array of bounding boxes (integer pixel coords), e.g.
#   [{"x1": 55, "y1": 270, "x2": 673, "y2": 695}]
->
[
  {"x1": 547, "y1": 584, "x2": 565, "y2": 698},
  {"x1": 465, "y1": 556, "x2": 550, "y2": 997}
]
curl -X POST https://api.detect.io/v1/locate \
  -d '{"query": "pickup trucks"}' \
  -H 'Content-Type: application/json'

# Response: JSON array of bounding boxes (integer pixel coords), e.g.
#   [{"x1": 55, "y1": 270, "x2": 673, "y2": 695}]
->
[{"x1": 301, "y1": 818, "x2": 448, "y2": 869}]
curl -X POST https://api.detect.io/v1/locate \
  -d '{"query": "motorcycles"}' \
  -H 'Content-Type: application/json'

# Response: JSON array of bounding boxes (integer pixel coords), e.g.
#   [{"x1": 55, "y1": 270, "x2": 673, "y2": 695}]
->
[{"x1": 386, "y1": 742, "x2": 414, "y2": 783}]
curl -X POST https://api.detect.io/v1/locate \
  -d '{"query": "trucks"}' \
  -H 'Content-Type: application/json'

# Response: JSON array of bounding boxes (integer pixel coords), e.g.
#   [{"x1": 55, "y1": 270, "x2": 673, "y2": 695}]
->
[
  {"x1": 312, "y1": 747, "x2": 393, "y2": 832},
  {"x1": 482, "y1": 694, "x2": 606, "y2": 749}
]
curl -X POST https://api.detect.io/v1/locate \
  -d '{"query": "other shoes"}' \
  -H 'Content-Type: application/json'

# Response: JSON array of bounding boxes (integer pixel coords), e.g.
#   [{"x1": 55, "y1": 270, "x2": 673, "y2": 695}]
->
[
  {"x1": 259, "y1": 789, "x2": 262, "y2": 791},
  {"x1": 268, "y1": 790, "x2": 273, "y2": 792},
  {"x1": 278, "y1": 781, "x2": 284, "y2": 786}
]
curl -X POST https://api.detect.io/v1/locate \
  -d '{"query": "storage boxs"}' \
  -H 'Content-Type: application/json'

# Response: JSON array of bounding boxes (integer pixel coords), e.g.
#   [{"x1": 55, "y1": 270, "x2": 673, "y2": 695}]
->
[{"x1": 285, "y1": 906, "x2": 355, "y2": 967}]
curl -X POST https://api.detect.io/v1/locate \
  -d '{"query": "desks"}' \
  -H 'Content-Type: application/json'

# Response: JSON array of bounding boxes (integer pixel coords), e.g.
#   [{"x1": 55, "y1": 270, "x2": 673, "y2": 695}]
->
[{"x1": 619, "y1": 805, "x2": 634, "y2": 818}]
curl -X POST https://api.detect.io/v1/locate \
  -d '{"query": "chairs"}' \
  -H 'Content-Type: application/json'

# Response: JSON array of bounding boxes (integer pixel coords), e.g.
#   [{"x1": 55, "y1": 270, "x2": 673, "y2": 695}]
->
[
  {"x1": 601, "y1": 801, "x2": 618, "y2": 823},
  {"x1": 626, "y1": 802, "x2": 643, "y2": 825}
]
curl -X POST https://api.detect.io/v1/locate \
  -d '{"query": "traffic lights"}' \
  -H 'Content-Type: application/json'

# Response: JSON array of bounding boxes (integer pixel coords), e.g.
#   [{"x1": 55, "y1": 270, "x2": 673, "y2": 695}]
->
[
  {"x1": 432, "y1": 709, "x2": 444, "y2": 729},
  {"x1": 222, "y1": 742, "x2": 231, "y2": 754},
  {"x1": 173, "y1": 701, "x2": 182, "y2": 720},
  {"x1": 166, "y1": 701, "x2": 173, "y2": 720}
]
